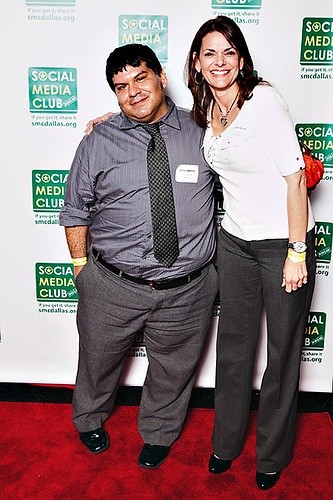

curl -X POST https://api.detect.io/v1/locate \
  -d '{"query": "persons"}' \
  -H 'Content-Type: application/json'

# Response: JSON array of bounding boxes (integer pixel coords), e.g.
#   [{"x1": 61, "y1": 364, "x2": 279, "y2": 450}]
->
[
  {"x1": 85, "y1": 16, "x2": 316, "y2": 491},
  {"x1": 59, "y1": 43, "x2": 219, "y2": 470}
]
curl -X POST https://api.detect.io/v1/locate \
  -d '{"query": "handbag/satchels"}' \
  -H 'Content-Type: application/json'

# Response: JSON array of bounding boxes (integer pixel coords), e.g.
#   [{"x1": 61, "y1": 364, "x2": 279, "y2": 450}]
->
[{"x1": 300, "y1": 142, "x2": 324, "y2": 196}]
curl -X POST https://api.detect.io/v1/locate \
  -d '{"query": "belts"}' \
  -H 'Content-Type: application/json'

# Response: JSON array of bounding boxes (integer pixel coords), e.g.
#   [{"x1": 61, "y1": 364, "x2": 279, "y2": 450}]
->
[{"x1": 91, "y1": 248, "x2": 213, "y2": 290}]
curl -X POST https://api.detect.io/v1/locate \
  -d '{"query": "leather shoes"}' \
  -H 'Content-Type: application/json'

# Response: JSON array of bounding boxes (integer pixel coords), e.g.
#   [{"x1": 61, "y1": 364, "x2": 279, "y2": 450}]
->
[
  {"x1": 79, "y1": 426, "x2": 110, "y2": 454},
  {"x1": 138, "y1": 443, "x2": 170, "y2": 469}
]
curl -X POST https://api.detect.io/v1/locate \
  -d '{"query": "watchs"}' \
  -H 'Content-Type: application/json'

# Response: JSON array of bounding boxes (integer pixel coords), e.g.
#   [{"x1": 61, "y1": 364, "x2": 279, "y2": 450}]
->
[{"x1": 288, "y1": 241, "x2": 307, "y2": 253}]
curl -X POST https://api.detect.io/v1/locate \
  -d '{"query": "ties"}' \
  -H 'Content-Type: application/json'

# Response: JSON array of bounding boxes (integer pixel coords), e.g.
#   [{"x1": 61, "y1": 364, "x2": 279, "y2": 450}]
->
[{"x1": 141, "y1": 121, "x2": 179, "y2": 269}]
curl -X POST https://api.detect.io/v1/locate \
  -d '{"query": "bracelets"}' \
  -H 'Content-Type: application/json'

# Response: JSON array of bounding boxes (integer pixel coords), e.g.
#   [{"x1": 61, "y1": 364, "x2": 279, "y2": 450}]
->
[
  {"x1": 287, "y1": 250, "x2": 306, "y2": 263},
  {"x1": 72, "y1": 258, "x2": 88, "y2": 266}
]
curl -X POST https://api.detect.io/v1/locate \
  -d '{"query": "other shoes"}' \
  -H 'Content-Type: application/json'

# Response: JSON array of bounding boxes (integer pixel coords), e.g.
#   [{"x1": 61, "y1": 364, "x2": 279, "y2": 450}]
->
[
  {"x1": 208, "y1": 452, "x2": 233, "y2": 474},
  {"x1": 255, "y1": 470, "x2": 281, "y2": 490}
]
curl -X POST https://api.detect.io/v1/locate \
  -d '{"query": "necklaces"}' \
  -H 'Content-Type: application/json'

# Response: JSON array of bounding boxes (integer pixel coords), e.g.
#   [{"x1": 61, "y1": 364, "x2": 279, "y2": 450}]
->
[{"x1": 218, "y1": 90, "x2": 240, "y2": 126}]
